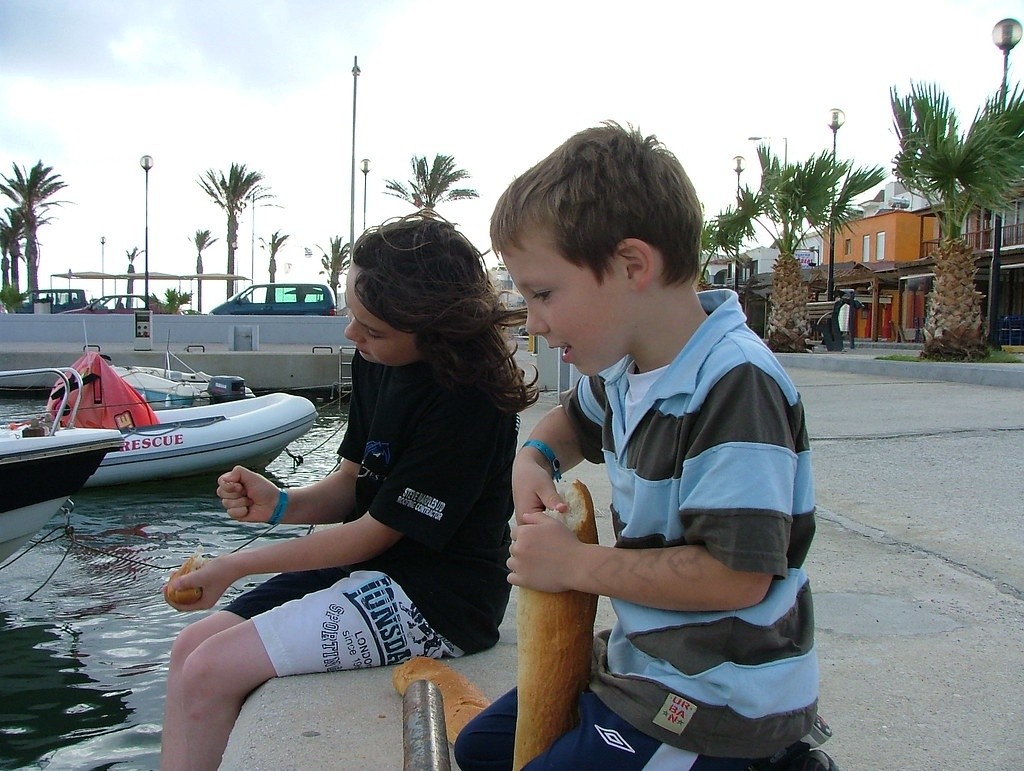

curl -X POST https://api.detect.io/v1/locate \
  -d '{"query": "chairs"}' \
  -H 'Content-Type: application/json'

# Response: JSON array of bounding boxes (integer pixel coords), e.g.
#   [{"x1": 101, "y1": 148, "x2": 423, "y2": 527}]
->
[
  {"x1": 914, "y1": 317, "x2": 926, "y2": 343},
  {"x1": 118, "y1": 303, "x2": 124, "y2": 308},
  {"x1": 996, "y1": 315, "x2": 1024, "y2": 346}
]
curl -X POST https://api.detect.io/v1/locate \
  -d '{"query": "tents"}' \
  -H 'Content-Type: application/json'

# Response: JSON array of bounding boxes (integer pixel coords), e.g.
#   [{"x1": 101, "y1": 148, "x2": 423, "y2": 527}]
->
[{"x1": 50, "y1": 271, "x2": 254, "y2": 310}]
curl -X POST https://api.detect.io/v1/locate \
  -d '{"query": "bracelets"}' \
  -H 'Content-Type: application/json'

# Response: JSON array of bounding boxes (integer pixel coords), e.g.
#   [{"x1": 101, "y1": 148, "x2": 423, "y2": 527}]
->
[
  {"x1": 521, "y1": 439, "x2": 562, "y2": 483},
  {"x1": 267, "y1": 487, "x2": 288, "y2": 525}
]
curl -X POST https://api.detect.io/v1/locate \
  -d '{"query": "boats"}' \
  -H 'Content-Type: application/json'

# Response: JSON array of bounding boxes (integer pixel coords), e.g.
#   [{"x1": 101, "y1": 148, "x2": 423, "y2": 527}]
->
[{"x1": 0, "y1": 318, "x2": 319, "y2": 562}]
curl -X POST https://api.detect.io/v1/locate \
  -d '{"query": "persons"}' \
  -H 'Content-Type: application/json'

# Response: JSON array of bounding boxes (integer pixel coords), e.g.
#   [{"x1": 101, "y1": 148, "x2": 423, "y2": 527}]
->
[
  {"x1": 160, "y1": 208, "x2": 538, "y2": 771},
  {"x1": 454, "y1": 120, "x2": 840, "y2": 771}
]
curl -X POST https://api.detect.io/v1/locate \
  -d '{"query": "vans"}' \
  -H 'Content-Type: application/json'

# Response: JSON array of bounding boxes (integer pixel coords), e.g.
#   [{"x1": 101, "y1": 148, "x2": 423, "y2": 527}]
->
[
  {"x1": 17, "y1": 289, "x2": 86, "y2": 314},
  {"x1": 209, "y1": 283, "x2": 338, "y2": 316}
]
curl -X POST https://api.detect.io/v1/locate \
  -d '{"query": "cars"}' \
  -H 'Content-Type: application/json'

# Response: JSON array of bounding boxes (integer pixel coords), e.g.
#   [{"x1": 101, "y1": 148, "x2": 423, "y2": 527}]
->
[
  {"x1": 518, "y1": 323, "x2": 529, "y2": 341},
  {"x1": 57, "y1": 295, "x2": 184, "y2": 314}
]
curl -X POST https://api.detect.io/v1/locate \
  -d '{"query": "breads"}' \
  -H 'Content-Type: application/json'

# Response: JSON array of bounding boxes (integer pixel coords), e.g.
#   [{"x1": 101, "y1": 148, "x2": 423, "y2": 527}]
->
[
  {"x1": 164, "y1": 555, "x2": 213, "y2": 605},
  {"x1": 393, "y1": 656, "x2": 493, "y2": 744},
  {"x1": 512, "y1": 479, "x2": 599, "y2": 771}
]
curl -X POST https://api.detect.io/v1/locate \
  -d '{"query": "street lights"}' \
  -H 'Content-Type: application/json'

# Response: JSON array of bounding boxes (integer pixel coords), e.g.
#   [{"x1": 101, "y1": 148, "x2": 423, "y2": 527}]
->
[
  {"x1": 100, "y1": 237, "x2": 106, "y2": 297},
  {"x1": 732, "y1": 156, "x2": 746, "y2": 293},
  {"x1": 747, "y1": 136, "x2": 787, "y2": 247},
  {"x1": 360, "y1": 158, "x2": 373, "y2": 231},
  {"x1": 140, "y1": 155, "x2": 153, "y2": 310},
  {"x1": 985, "y1": 19, "x2": 1023, "y2": 350},
  {"x1": 826, "y1": 109, "x2": 845, "y2": 301}
]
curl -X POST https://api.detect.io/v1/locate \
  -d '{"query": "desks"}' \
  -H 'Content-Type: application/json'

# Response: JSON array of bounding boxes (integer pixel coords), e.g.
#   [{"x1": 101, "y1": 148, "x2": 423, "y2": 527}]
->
[{"x1": 904, "y1": 328, "x2": 918, "y2": 343}]
